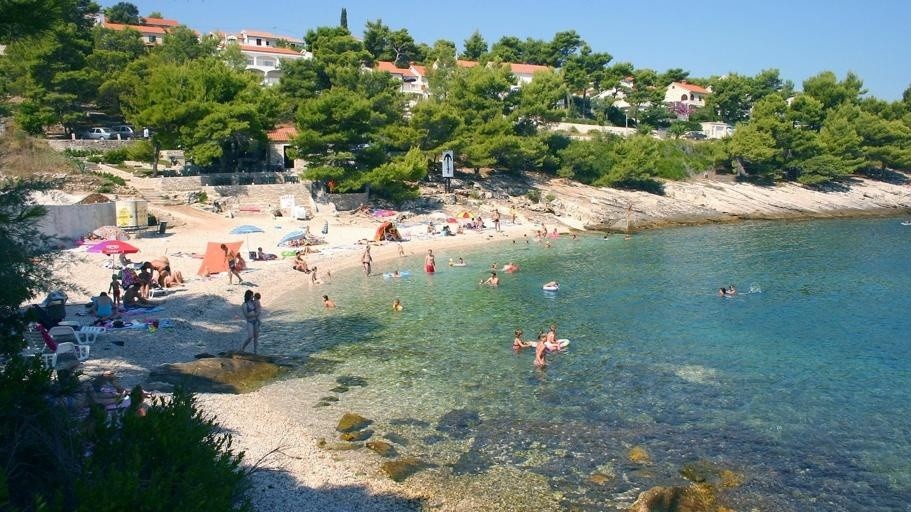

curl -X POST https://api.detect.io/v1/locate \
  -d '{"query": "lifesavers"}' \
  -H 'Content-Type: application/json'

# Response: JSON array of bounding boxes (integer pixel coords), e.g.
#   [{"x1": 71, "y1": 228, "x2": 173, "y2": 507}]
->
[
  {"x1": 543, "y1": 286, "x2": 557, "y2": 291},
  {"x1": 547, "y1": 339, "x2": 569, "y2": 350},
  {"x1": 383, "y1": 272, "x2": 404, "y2": 278}
]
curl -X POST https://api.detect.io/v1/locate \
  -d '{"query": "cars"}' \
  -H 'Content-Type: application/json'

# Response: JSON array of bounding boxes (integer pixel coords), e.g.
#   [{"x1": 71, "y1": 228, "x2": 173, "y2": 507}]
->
[{"x1": 70, "y1": 125, "x2": 153, "y2": 139}]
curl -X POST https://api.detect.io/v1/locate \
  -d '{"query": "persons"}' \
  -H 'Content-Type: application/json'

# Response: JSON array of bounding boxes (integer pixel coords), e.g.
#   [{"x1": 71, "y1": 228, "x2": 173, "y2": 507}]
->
[
  {"x1": 513, "y1": 328, "x2": 533, "y2": 352},
  {"x1": 328, "y1": 178, "x2": 338, "y2": 195},
  {"x1": 87, "y1": 243, "x2": 264, "y2": 361},
  {"x1": 720, "y1": 287, "x2": 727, "y2": 298},
  {"x1": 729, "y1": 284, "x2": 736, "y2": 295},
  {"x1": 547, "y1": 324, "x2": 561, "y2": 351},
  {"x1": 231, "y1": 204, "x2": 581, "y2": 291},
  {"x1": 534, "y1": 330, "x2": 550, "y2": 367}
]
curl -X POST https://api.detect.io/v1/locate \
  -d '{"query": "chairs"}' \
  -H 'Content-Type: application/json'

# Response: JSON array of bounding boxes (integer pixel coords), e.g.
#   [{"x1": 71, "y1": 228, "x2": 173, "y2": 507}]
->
[
  {"x1": 138, "y1": 286, "x2": 154, "y2": 300},
  {"x1": 119, "y1": 253, "x2": 144, "y2": 271},
  {"x1": 17, "y1": 304, "x2": 106, "y2": 376}
]
